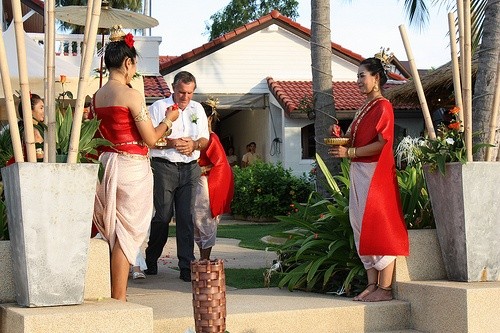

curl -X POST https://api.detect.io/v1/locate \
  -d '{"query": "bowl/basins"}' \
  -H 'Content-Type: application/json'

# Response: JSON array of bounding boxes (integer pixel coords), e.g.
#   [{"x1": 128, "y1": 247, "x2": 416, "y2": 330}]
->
[{"x1": 324, "y1": 138, "x2": 350, "y2": 146}]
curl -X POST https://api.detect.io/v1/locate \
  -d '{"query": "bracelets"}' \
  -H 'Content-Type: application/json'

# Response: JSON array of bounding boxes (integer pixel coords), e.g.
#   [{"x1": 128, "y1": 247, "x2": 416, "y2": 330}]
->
[
  {"x1": 159, "y1": 118, "x2": 173, "y2": 131},
  {"x1": 347, "y1": 147, "x2": 357, "y2": 158}
]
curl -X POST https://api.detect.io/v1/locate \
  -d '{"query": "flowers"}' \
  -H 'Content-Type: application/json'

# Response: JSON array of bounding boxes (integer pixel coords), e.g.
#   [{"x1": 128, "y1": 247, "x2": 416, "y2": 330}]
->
[
  {"x1": 34, "y1": 74, "x2": 116, "y2": 186},
  {"x1": 411, "y1": 104, "x2": 498, "y2": 179},
  {"x1": 188, "y1": 113, "x2": 199, "y2": 125},
  {"x1": 123, "y1": 32, "x2": 135, "y2": 50}
]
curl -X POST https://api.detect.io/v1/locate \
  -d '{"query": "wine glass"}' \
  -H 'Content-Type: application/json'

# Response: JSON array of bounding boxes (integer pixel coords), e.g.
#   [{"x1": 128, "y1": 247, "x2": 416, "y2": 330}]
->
[{"x1": 154, "y1": 128, "x2": 173, "y2": 146}]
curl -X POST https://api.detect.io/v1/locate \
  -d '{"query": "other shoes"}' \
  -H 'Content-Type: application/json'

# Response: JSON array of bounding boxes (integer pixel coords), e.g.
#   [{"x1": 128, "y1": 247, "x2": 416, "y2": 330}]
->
[
  {"x1": 133, "y1": 272, "x2": 146, "y2": 280},
  {"x1": 143, "y1": 269, "x2": 157, "y2": 275},
  {"x1": 179, "y1": 269, "x2": 191, "y2": 282}
]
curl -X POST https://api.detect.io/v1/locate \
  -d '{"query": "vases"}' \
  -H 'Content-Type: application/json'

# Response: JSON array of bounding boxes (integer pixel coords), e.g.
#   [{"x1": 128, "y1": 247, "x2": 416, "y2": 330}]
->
[
  {"x1": 420, "y1": 160, "x2": 500, "y2": 282},
  {"x1": 0, "y1": 160, "x2": 101, "y2": 309}
]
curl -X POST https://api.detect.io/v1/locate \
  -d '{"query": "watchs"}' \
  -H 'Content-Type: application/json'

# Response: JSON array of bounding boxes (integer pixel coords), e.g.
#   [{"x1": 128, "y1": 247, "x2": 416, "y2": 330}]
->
[{"x1": 196, "y1": 140, "x2": 201, "y2": 150}]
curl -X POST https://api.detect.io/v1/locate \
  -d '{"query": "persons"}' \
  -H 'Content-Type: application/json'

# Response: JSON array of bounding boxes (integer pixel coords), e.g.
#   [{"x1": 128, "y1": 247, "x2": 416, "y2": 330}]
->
[
  {"x1": 226, "y1": 143, "x2": 261, "y2": 169},
  {"x1": 18, "y1": 93, "x2": 45, "y2": 162},
  {"x1": 87, "y1": 25, "x2": 180, "y2": 302},
  {"x1": 145, "y1": 70, "x2": 210, "y2": 282},
  {"x1": 191, "y1": 96, "x2": 231, "y2": 274},
  {"x1": 327, "y1": 57, "x2": 409, "y2": 303},
  {"x1": 132, "y1": 203, "x2": 155, "y2": 278}
]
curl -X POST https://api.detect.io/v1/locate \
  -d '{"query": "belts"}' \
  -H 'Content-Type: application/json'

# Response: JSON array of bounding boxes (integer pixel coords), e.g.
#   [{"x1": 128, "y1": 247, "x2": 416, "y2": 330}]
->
[{"x1": 152, "y1": 157, "x2": 198, "y2": 167}]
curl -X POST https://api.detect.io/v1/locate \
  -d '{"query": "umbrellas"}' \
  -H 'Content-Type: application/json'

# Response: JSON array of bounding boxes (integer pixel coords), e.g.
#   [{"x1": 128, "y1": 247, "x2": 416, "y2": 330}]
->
[{"x1": 54, "y1": 1, "x2": 159, "y2": 89}]
